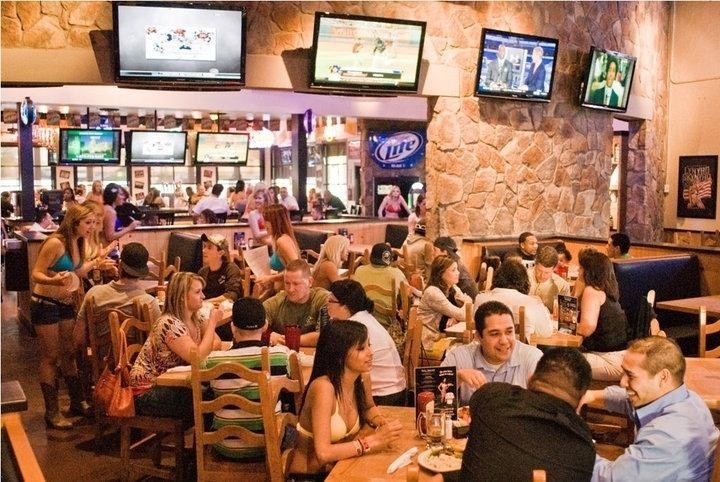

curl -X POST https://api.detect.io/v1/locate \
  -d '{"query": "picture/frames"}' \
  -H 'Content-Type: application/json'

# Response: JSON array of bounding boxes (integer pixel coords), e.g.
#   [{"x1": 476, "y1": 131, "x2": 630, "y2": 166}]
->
[{"x1": 677, "y1": 155, "x2": 718, "y2": 219}]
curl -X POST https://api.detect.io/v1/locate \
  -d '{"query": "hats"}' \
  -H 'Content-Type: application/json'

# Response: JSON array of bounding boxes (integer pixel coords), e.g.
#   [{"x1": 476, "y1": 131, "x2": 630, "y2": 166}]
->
[
  {"x1": 200, "y1": 232, "x2": 229, "y2": 256},
  {"x1": 413, "y1": 218, "x2": 426, "y2": 233},
  {"x1": 432, "y1": 235, "x2": 462, "y2": 261},
  {"x1": 120, "y1": 243, "x2": 150, "y2": 278},
  {"x1": 369, "y1": 242, "x2": 392, "y2": 265},
  {"x1": 229, "y1": 296, "x2": 267, "y2": 330}
]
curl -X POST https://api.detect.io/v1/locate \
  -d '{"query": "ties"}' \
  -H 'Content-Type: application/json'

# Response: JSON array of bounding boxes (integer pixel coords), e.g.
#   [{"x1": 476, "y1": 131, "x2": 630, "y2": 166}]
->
[{"x1": 605, "y1": 93, "x2": 609, "y2": 106}]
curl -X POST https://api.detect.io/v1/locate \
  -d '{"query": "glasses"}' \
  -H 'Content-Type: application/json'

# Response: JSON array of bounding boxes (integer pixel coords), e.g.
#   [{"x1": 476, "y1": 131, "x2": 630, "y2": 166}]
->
[
  {"x1": 328, "y1": 297, "x2": 341, "y2": 304},
  {"x1": 414, "y1": 224, "x2": 426, "y2": 231}
]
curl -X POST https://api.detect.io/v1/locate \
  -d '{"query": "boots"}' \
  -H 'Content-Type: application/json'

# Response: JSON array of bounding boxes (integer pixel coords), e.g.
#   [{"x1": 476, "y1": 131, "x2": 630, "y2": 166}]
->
[
  {"x1": 61, "y1": 370, "x2": 94, "y2": 419},
  {"x1": 38, "y1": 379, "x2": 75, "y2": 431}
]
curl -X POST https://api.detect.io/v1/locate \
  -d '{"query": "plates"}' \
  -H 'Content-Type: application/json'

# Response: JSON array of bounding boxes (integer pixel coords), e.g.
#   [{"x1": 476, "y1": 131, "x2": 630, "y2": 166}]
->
[{"x1": 417, "y1": 444, "x2": 463, "y2": 474}]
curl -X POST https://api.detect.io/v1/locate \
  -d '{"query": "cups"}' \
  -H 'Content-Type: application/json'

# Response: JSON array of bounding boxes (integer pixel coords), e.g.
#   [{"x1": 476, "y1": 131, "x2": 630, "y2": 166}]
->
[
  {"x1": 260, "y1": 324, "x2": 272, "y2": 347},
  {"x1": 559, "y1": 266, "x2": 568, "y2": 278},
  {"x1": 415, "y1": 409, "x2": 445, "y2": 450},
  {"x1": 285, "y1": 325, "x2": 301, "y2": 353}
]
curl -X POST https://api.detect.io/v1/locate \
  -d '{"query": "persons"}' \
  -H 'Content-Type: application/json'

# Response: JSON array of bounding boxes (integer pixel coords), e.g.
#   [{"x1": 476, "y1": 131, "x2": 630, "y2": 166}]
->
[
  {"x1": 328, "y1": 65, "x2": 340, "y2": 81},
  {"x1": 590, "y1": 58, "x2": 619, "y2": 106},
  {"x1": 519, "y1": 46, "x2": 546, "y2": 92},
  {"x1": 486, "y1": 45, "x2": 514, "y2": 91},
  {"x1": 371, "y1": 37, "x2": 387, "y2": 57},
  {"x1": 352, "y1": 37, "x2": 364, "y2": 65},
  {"x1": 3, "y1": 181, "x2": 718, "y2": 482}
]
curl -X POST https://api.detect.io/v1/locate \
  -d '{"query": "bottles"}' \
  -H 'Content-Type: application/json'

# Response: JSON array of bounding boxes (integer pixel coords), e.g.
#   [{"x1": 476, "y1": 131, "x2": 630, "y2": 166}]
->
[
  {"x1": 113, "y1": 259, "x2": 121, "y2": 283},
  {"x1": 111, "y1": 246, "x2": 120, "y2": 260},
  {"x1": 92, "y1": 258, "x2": 103, "y2": 285},
  {"x1": 444, "y1": 416, "x2": 453, "y2": 440},
  {"x1": 349, "y1": 233, "x2": 354, "y2": 244},
  {"x1": 416, "y1": 392, "x2": 435, "y2": 438}
]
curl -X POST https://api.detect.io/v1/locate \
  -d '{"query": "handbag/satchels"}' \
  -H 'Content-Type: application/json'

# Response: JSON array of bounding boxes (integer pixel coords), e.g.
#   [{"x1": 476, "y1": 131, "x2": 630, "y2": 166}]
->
[
  {"x1": 384, "y1": 320, "x2": 405, "y2": 358},
  {"x1": 91, "y1": 365, "x2": 136, "y2": 419}
]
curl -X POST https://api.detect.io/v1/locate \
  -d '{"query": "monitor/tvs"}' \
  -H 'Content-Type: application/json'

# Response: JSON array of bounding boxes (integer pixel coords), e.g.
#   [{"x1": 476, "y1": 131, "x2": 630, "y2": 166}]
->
[
  {"x1": 194, "y1": 132, "x2": 249, "y2": 166},
  {"x1": 126, "y1": 127, "x2": 188, "y2": 166},
  {"x1": 474, "y1": 26, "x2": 559, "y2": 102},
  {"x1": 580, "y1": 44, "x2": 637, "y2": 114},
  {"x1": 111, "y1": 2, "x2": 247, "y2": 89},
  {"x1": 308, "y1": 11, "x2": 427, "y2": 94},
  {"x1": 58, "y1": 129, "x2": 122, "y2": 167}
]
